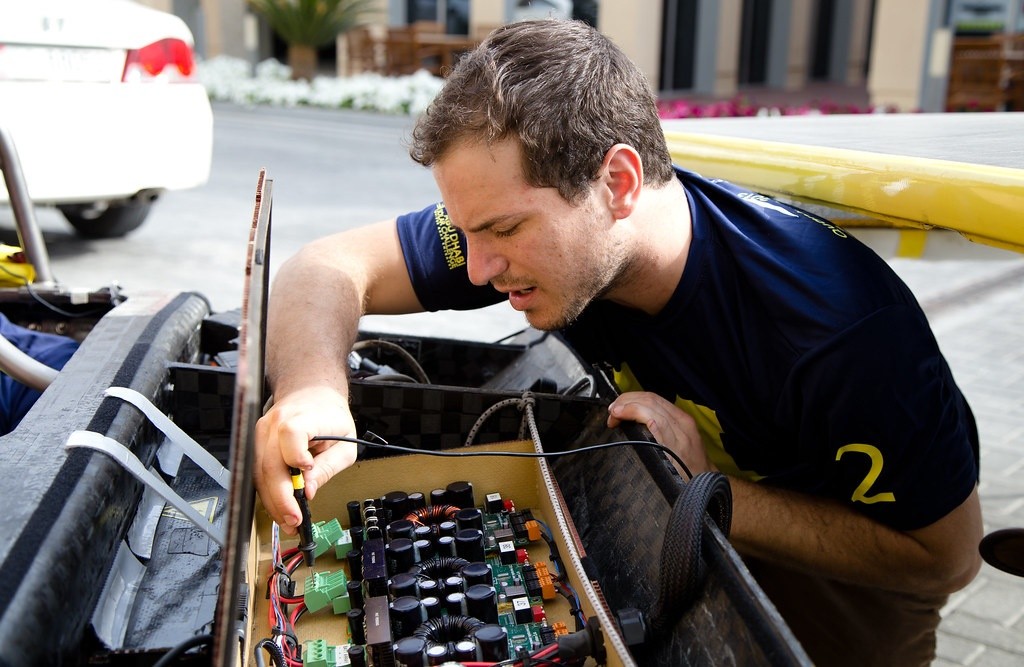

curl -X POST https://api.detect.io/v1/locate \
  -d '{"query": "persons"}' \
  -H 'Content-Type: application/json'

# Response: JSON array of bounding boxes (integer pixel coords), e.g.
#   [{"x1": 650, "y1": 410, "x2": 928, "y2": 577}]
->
[
  {"x1": 254, "y1": 18, "x2": 985, "y2": 667},
  {"x1": 0, "y1": 313, "x2": 79, "y2": 432}
]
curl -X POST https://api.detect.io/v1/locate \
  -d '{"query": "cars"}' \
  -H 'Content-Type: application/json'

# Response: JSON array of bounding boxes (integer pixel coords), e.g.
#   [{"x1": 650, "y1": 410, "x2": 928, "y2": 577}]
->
[{"x1": 0, "y1": 3, "x2": 216, "y2": 241}]
